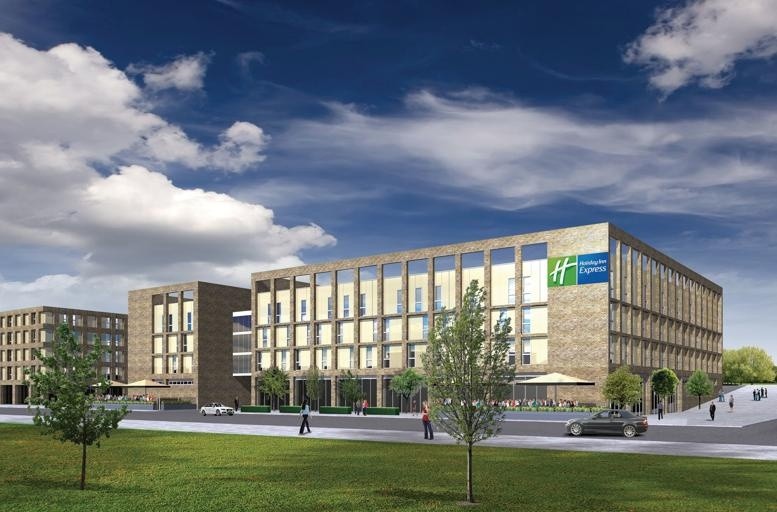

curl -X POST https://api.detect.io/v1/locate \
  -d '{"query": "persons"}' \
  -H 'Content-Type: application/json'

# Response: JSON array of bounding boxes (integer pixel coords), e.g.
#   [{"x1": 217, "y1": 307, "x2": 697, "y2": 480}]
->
[
  {"x1": 656, "y1": 400, "x2": 663, "y2": 421},
  {"x1": 297, "y1": 399, "x2": 311, "y2": 435},
  {"x1": 434, "y1": 398, "x2": 578, "y2": 410},
  {"x1": 709, "y1": 401, "x2": 715, "y2": 421},
  {"x1": 717, "y1": 388, "x2": 721, "y2": 402},
  {"x1": 96, "y1": 393, "x2": 158, "y2": 402},
  {"x1": 355, "y1": 399, "x2": 368, "y2": 416},
  {"x1": 412, "y1": 400, "x2": 416, "y2": 416},
  {"x1": 420, "y1": 399, "x2": 433, "y2": 440},
  {"x1": 233, "y1": 395, "x2": 239, "y2": 412},
  {"x1": 752, "y1": 387, "x2": 767, "y2": 401},
  {"x1": 721, "y1": 390, "x2": 725, "y2": 402},
  {"x1": 728, "y1": 394, "x2": 734, "y2": 412}
]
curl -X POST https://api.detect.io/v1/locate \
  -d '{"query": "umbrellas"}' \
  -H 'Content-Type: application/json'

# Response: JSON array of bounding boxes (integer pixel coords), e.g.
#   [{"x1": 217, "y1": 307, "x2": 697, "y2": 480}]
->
[
  {"x1": 515, "y1": 373, "x2": 594, "y2": 401},
  {"x1": 439, "y1": 375, "x2": 507, "y2": 400},
  {"x1": 121, "y1": 378, "x2": 170, "y2": 394},
  {"x1": 91, "y1": 380, "x2": 126, "y2": 388}
]
